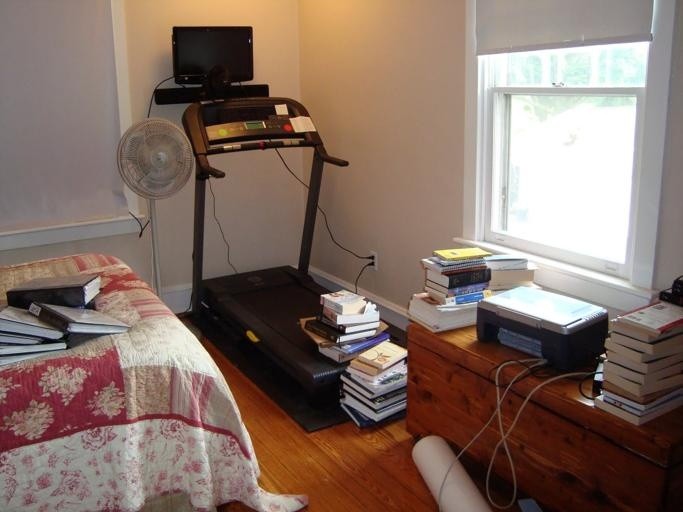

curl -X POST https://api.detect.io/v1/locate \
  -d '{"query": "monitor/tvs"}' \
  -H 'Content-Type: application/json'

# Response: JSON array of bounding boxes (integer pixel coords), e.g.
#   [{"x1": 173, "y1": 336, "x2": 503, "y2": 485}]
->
[{"x1": 172, "y1": 26, "x2": 254, "y2": 85}]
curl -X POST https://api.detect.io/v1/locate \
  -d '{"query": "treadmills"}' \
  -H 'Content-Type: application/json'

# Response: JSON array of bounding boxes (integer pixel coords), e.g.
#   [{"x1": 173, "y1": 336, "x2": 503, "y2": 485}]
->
[{"x1": 153, "y1": 26, "x2": 408, "y2": 394}]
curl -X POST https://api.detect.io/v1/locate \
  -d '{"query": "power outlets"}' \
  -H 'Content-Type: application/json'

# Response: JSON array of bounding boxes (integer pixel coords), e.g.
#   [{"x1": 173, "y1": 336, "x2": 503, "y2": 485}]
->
[{"x1": 367, "y1": 248, "x2": 378, "y2": 272}]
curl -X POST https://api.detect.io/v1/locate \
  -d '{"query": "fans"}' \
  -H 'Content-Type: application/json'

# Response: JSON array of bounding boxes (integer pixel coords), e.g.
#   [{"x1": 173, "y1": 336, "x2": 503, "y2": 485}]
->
[{"x1": 114, "y1": 115, "x2": 203, "y2": 343}]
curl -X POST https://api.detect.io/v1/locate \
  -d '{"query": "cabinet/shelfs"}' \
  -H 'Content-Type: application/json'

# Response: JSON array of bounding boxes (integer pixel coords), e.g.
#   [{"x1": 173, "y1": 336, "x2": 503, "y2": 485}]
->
[{"x1": 402, "y1": 301, "x2": 682, "y2": 512}]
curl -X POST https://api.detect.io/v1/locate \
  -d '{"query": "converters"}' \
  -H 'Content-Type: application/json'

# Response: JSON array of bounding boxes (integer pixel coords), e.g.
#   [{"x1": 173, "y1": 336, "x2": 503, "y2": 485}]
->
[{"x1": 592, "y1": 361, "x2": 604, "y2": 394}]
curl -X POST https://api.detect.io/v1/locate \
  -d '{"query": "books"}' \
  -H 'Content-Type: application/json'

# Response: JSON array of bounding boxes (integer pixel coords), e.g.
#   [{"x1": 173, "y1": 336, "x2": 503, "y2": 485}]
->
[
  {"x1": 0, "y1": 305, "x2": 69, "y2": 341},
  {"x1": 0, "y1": 352, "x2": 48, "y2": 367},
  {"x1": 28, "y1": 300, "x2": 131, "y2": 335},
  {"x1": 592, "y1": 293, "x2": 683, "y2": 430},
  {"x1": 4, "y1": 272, "x2": 101, "y2": 308},
  {"x1": 0, "y1": 333, "x2": 43, "y2": 346},
  {"x1": 0, "y1": 341, "x2": 68, "y2": 357},
  {"x1": 295, "y1": 287, "x2": 408, "y2": 433},
  {"x1": 407, "y1": 246, "x2": 542, "y2": 335}
]
